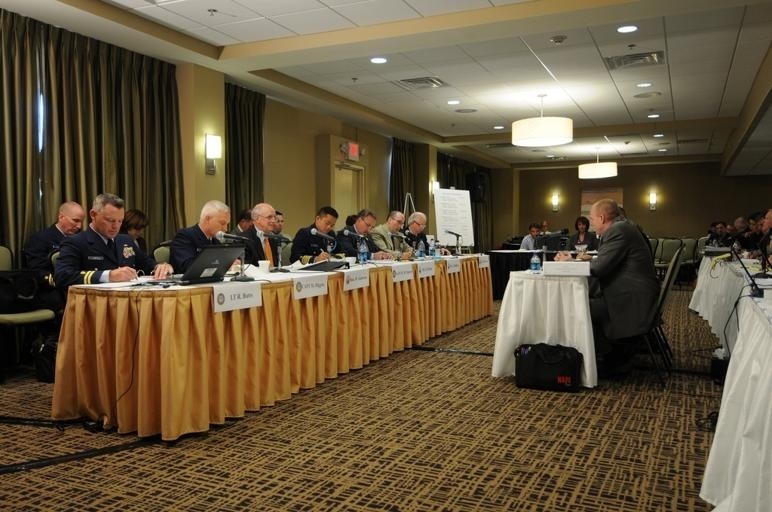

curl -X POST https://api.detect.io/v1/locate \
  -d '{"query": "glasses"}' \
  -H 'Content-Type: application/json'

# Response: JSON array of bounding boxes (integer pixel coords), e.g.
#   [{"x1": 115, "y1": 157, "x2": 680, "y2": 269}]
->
[
  {"x1": 389, "y1": 216, "x2": 404, "y2": 226},
  {"x1": 413, "y1": 220, "x2": 426, "y2": 228},
  {"x1": 359, "y1": 216, "x2": 374, "y2": 232},
  {"x1": 257, "y1": 214, "x2": 279, "y2": 223}
]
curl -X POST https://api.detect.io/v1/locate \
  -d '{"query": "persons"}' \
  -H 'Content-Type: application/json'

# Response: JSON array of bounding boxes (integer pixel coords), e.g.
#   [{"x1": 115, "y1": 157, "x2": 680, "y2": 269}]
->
[
  {"x1": 337, "y1": 209, "x2": 395, "y2": 260},
  {"x1": 521, "y1": 207, "x2": 654, "y2": 267},
  {"x1": 54, "y1": 192, "x2": 174, "y2": 287},
  {"x1": 167, "y1": 201, "x2": 242, "y2": 274},
  {"x1": 403, "y1": 212, "x2": 452, "y2": 256},
  {"x1": 706, "y1": 209, "x2": 772, "y2": 274},
  {"x1": 24, "y1": 201, "x2": 86, "y2": 284},
  {"x1": 232, "y1": 209, "x2": 285, "y2": 243},
  {"x1": 120, "y1": 208, "x2": 149, "y2": 253},
  {"x1": 290, "y1": 206, "x2": 348, "y2": 264},
  {"x1": 371, "y1": 210, "x2": 413, "y2": 261},
  {"x1": 554, "y1": 198, "x2": 658, "y2": 333},
  {"x1": 234, "y1": 203, "x2": 279, "y2": 268}
]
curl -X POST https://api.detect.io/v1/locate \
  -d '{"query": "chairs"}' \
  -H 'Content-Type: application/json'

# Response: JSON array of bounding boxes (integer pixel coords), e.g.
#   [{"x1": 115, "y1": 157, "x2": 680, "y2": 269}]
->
[
  {"x1": 610, "y1": 234, "x2": 709, "y2": 388},
  {"x1": 0, "y1": 244, "x2": 58, "y2": 387}
]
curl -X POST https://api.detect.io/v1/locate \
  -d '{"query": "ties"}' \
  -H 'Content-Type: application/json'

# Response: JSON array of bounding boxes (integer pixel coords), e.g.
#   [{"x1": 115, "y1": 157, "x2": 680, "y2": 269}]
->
[
  {"x1": 264, "y1": 238, "x2": 274, "y2": 269},
  {"x1": 543, "y1": 232, "x2": 546, "y2": 235}
]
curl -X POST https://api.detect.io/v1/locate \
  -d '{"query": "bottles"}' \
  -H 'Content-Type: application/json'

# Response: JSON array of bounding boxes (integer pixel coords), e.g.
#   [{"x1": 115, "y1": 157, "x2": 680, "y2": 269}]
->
[
  {"x1": 531, "y1": 251, "x2": 540, "y2": 274},
  {"x1": 428, "y1": 238, "x2": 435, "y2": 255},
  {"x1": 357, "y1": 237, "x2": 367, "y2": 264},
  {"x1": 417, "y1": 238, "x2": 426, "y2": 257}
]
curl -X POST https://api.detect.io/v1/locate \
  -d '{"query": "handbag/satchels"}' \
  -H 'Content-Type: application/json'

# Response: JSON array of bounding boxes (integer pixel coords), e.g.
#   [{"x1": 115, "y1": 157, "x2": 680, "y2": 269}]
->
[
  {"x1": 1, "y1": 269, "x2": 43, "y2": 314},
  {"x1": 513, "y1": 343, "x2": 583, "y2": 391}
]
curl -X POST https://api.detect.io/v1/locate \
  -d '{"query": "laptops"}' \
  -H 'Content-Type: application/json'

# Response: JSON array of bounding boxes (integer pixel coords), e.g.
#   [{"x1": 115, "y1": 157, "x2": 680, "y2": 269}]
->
[{"x1": 149, "y1": 243, "x2": 247, "y2": 286}]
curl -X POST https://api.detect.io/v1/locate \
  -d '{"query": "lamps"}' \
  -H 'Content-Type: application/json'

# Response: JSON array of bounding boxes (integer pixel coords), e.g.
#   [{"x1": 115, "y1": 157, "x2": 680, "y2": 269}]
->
[
  {"x1": 511, "y1": 97, "x2": 574, "y2": 147},
  {"x1": 549, "y1": 190, "x2": 559, "y2": 212},
  {"x1": 204, "y1": 134, "x2": 225, "y2": 179},
  {"x1": 648, "y1": 190, "x2": 657, "y2": 211},
  {"x1": 577, "y1": 146, "x2": 618, "y2": 180}
]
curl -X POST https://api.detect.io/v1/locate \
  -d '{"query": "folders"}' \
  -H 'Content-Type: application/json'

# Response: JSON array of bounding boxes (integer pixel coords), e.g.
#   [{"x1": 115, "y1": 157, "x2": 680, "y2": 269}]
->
[{"x1": 297, "y1": 260, "x2": 350, "y2": 272}]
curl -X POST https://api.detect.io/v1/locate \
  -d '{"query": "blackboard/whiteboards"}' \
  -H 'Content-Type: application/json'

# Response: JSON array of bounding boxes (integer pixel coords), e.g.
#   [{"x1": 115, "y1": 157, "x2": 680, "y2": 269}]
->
[{"x1": 434, "y1": 188, "x2": 475, "y2": 247}]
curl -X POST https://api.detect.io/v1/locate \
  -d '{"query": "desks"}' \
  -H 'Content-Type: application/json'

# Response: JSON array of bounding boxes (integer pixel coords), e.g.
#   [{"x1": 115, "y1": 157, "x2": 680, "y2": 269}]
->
[
  {"x1": 51, "y1": 254, "x2": 495, "y2": 442},
  {"x1": 490, "y1": 249, "x2": 601, "y2": 389},
  {"x1": 691, "y1": 236, "x2": 772, "y2": 512}
]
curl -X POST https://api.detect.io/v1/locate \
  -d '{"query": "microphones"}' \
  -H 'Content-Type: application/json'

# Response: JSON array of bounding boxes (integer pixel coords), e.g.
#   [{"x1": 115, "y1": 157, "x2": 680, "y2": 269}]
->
[
  {"x1": 445, "y1": 230, "x2": 461, "y2": 236},
  {"x1": 217, "y1": 231, "x2": 252, "y2": 243},
  {"x1": 344, "y1": 230, "x2": 368, "y2": 239},
  {"x1": 257, "y1": 230, "x2": 288, "y2": 240},
  {"x1": 405, "y1": 230, "x2": 419, "y2": 239},
  {"x1": 310, "y1": 228, "x2": 336, "y2": 241}
]
